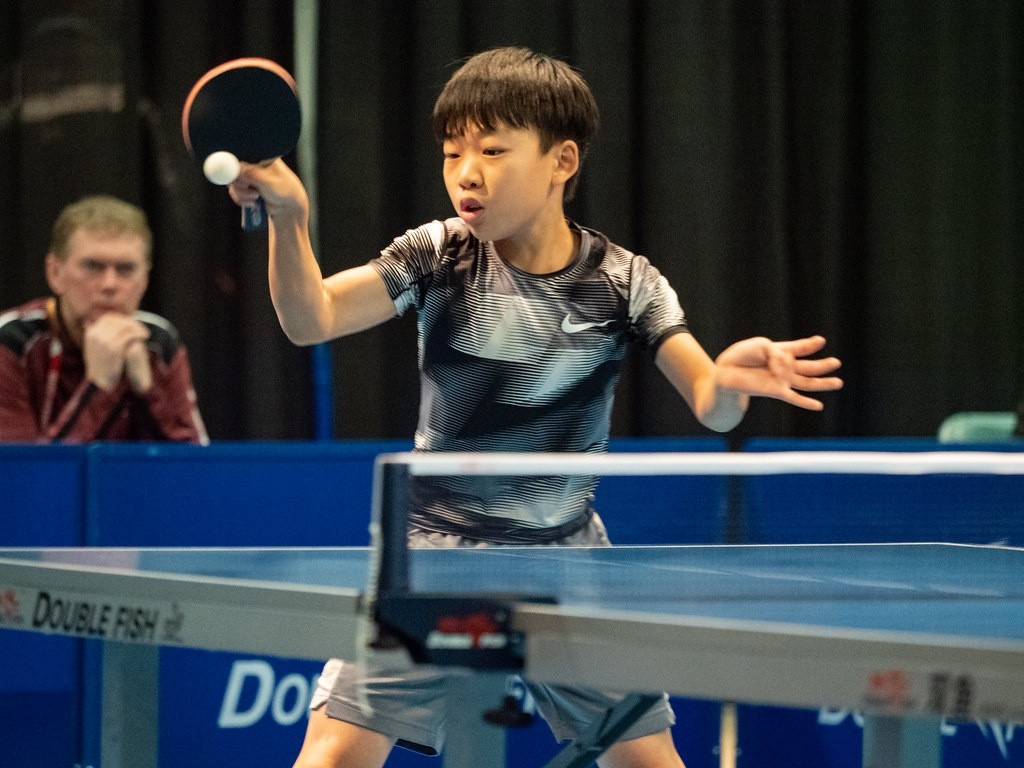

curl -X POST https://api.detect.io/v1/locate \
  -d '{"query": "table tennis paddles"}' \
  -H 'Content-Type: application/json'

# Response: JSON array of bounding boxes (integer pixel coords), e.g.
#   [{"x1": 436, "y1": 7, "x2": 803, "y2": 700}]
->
[{"x1": 182, "y1": 58, "x2": 303, "y2": 228}]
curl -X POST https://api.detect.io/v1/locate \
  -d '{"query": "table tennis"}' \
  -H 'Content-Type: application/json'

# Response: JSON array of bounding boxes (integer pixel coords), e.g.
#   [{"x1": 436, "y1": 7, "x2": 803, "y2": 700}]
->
[{"x1": 204, "y1": 151, "x2": 241, "y2": 185}]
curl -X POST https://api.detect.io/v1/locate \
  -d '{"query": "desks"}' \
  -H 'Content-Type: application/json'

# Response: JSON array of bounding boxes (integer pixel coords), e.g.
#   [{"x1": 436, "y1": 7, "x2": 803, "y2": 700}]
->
[{"x1": 1, "y1": 541, "x2": 1024, "y2": 767}]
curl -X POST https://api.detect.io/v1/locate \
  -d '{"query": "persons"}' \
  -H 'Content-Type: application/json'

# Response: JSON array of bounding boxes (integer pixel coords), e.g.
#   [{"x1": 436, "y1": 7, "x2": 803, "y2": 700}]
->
[
  {"x1": 230, "y1": 47, "x2": 843, "y2": 768},
  {"x1": 0, "y1": 197, "x2": 209, "y2": 446}
]
